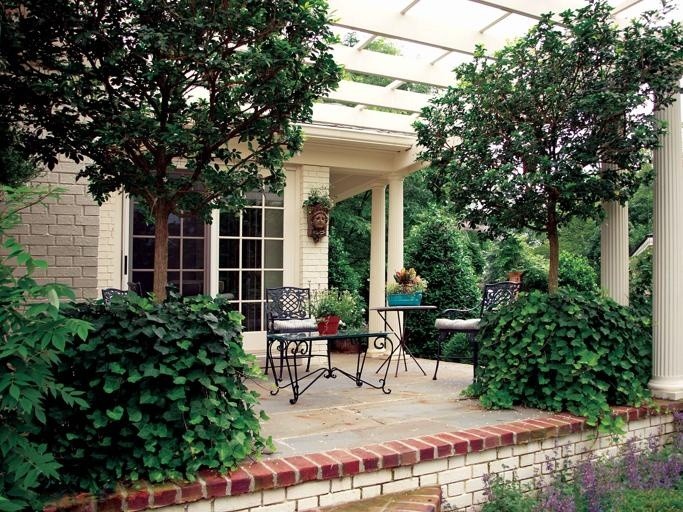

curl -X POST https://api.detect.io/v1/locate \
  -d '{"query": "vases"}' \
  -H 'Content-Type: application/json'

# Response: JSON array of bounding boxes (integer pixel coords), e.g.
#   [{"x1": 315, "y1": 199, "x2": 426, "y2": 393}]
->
[{"x1": 387, "y1": 290, "x2": 424, "y2": 307}]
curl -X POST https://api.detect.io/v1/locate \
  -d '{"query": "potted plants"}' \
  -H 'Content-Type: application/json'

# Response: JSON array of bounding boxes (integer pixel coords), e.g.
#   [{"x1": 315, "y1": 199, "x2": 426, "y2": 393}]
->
[{"x1": 305, "y1": 287, "x2": 364, "y2": 335}]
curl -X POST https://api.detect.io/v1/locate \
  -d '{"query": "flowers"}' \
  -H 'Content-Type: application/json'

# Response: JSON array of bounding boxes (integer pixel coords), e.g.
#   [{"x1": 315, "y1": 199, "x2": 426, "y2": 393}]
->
[{"x1": 385, "y1": 268, "x2": 426, "y2": 296}]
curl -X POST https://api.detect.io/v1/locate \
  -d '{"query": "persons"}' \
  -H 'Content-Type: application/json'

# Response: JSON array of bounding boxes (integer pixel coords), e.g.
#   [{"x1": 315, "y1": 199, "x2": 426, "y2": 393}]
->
[{"x1": 310, "y1": 211, "x2": 329, "y2": 235}]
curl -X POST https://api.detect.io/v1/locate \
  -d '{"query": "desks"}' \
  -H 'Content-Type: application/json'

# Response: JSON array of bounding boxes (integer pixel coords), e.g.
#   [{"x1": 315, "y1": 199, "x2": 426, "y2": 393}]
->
[
  {"x1": 266, "y1": 330, "x2": 393, "y2": 403},
  {"x1": 368, "y1": 306, "x2": 436, "y2": 378}
]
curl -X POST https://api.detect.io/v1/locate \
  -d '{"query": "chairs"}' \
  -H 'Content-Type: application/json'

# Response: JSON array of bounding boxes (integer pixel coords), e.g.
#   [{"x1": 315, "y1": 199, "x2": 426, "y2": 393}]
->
[
  {"x1": 261, "y1": 283, "x2": 345, "y2": 383},
  {"x1": 431, "y1": 281, "x2": 524, "y2": 388}
]
